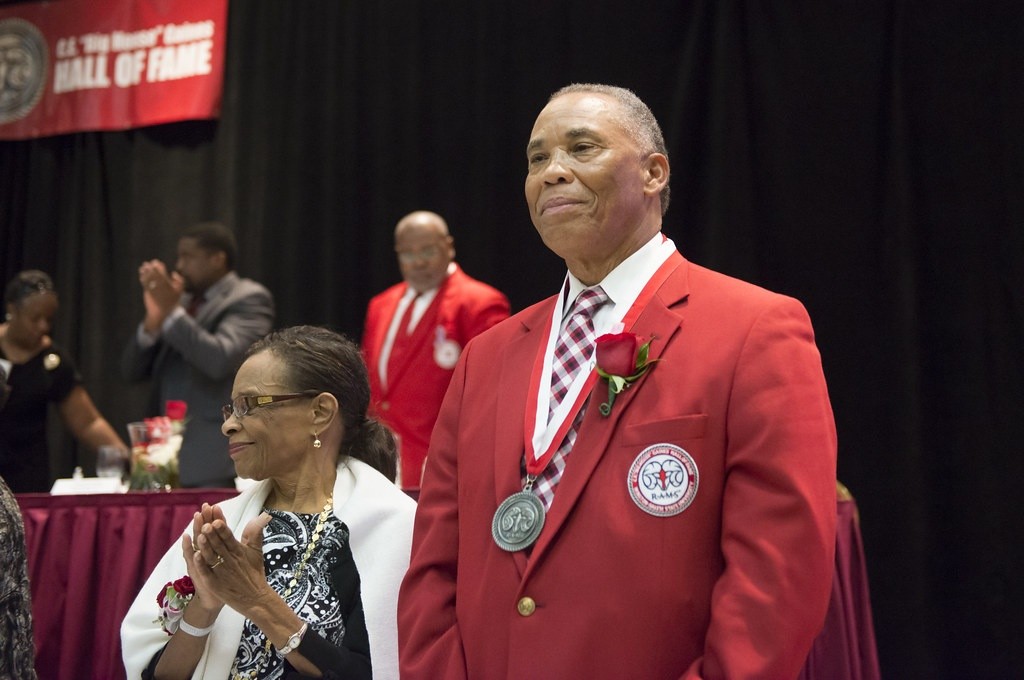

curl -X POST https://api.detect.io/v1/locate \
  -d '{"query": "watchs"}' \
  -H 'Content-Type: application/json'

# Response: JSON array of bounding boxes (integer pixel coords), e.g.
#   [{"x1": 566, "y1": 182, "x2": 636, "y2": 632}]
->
[{"x1": 275, "y1": 622, "x2": 308, "y2": 659}]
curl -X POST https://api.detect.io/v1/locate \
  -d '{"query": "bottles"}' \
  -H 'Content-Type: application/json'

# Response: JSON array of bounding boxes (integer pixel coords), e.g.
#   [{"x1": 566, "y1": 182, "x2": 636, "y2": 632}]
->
[{"x1": 72, "y1": 467, "x2": 84, "y2": 479}]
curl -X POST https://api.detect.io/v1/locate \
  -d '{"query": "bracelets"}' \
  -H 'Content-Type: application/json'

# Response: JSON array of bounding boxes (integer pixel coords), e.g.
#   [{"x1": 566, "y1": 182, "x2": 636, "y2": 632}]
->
[{"x1": 179, "y1": 618, "x2": 215, "y2": 637}]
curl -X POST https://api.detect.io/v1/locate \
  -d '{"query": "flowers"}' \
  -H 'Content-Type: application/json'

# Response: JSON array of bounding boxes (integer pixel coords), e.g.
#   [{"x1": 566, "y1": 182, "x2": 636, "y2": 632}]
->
[
  {"x1": 590, "y1": 332, "x2": 667, "y2": 416},
  {"x1": 127, "y1": 399, "x2": 188, "y2": 492}
]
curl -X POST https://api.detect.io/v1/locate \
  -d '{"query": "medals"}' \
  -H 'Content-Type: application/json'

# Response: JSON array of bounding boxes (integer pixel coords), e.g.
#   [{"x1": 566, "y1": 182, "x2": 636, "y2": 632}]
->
[{"x1": 492, "y1": 491, "x2": 546, "y2": 552}]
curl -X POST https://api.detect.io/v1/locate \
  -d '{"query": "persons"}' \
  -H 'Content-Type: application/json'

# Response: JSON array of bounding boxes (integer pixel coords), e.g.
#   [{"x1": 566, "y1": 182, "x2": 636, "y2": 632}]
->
[
  {"x1": 0, "y1": 477, "x2": 36, "y2": 680},
  {"x1": 120, "y1": 326, "x2": 418, "y2": 680},
  {"x1": 0, "y1": 271, "x2": 132, "y2": 493},
  {"x1": 120, "y1": 221, "x2": 274, "y2": 489},
  {"x1": 362, "y1": 211, "x2": 511, "y2": 489},
  {"x1": 395, "y1": 83, "x2": 837, "y2": 680}
]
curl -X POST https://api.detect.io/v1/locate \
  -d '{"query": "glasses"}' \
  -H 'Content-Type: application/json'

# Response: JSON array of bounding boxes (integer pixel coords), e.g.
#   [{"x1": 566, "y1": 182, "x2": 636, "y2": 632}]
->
[{"x1": 222, "y1": 389, "x2": 320, "y2": 422}]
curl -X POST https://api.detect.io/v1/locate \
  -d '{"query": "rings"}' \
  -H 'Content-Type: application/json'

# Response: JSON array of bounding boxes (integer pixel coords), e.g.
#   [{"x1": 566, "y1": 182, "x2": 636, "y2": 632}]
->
[
  {"x1": 149, "y1": 282, "x2": 156, "y2": 288},
  {"x1": 211, "y1": 555, "x2": 224, "y2": 568}
]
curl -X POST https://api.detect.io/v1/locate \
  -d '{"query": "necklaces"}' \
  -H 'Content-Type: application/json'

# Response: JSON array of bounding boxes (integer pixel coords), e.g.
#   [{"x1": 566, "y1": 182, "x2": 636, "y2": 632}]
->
[{"x1": 230, "y1": 492, "x2": 333, "y2": 680}]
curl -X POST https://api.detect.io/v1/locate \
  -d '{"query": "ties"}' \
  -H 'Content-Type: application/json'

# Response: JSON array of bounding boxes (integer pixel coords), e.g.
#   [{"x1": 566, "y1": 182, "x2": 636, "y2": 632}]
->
[
  {"x1": 521, "y1": 285, "x2": 609, "y2": 514},
  {"x1": 387, "y1": 290, "x2": 425, "y2": 388}
]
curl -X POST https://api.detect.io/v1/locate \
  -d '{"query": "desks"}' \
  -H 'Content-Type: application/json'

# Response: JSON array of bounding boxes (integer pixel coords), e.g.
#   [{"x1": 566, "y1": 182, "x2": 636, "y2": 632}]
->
[{"x1": 11, "y1": 483, "x2": 880, "y2": 680}]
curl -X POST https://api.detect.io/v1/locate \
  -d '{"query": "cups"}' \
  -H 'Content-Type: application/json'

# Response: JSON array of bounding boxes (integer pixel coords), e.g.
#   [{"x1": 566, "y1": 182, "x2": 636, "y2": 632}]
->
[{"x1": 95, "y1": 447, "x2": 124, "y2": 479}]
